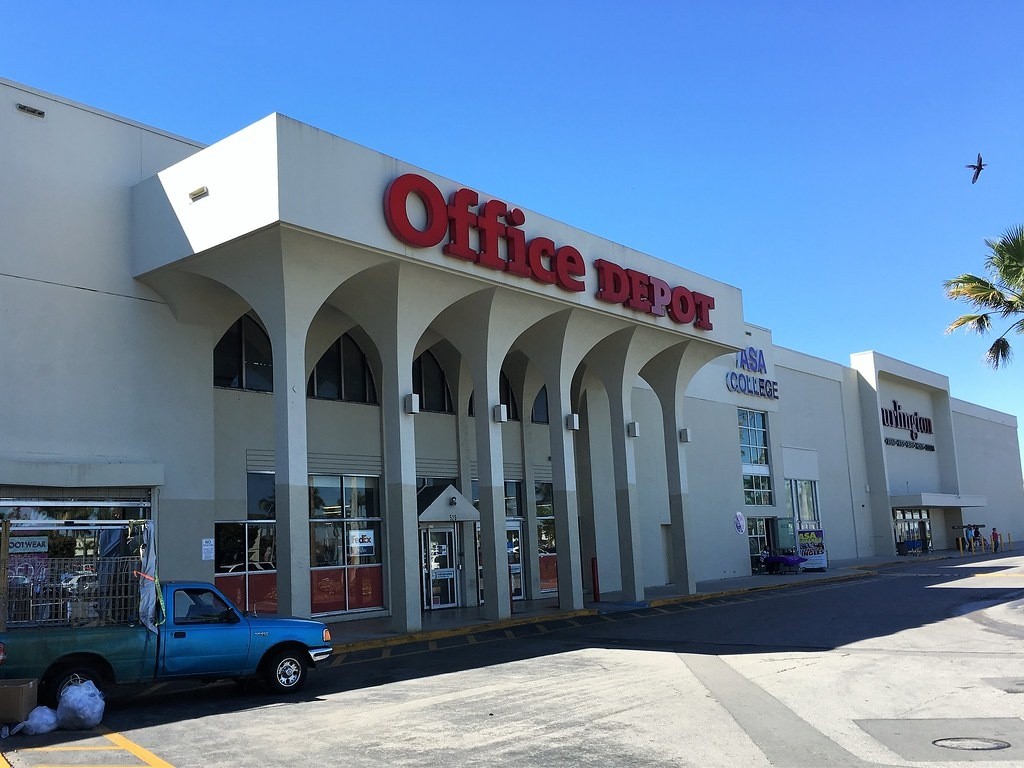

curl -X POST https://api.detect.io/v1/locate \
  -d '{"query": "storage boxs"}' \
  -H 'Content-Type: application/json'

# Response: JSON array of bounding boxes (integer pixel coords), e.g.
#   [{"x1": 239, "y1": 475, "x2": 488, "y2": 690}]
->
[{"x1": 0, "y1": 680, "x2": 37, "y2": 723}]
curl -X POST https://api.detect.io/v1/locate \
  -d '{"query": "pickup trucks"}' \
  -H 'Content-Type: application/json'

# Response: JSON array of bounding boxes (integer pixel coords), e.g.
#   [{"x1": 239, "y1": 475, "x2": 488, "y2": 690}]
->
[{"x1": 0, "y1": 581, "x2": 335, "y2": 711}]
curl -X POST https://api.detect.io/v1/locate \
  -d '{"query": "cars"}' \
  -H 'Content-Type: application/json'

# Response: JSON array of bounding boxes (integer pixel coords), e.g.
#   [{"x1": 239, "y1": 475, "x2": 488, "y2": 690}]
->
[
  {"x1": 424, "y1": 553, "x2": 448, "y2": 575},
  {"x1": 7, "y1": 574, "x2": 98, "y2": 595}
]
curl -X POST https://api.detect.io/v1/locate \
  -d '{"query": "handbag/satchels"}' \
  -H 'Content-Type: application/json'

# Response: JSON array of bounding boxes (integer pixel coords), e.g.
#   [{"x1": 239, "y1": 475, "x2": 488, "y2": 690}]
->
[
  {"x1": 11, "y1": 706, "x2": 58, "y2": 736},
  {"x1": 57, "y1": 679, "x2": 105, "y2": 729}
]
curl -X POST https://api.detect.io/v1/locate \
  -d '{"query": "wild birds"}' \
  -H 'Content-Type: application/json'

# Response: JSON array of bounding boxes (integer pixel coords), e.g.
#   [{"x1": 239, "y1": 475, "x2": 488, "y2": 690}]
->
[{"x1": 965, "y1": 153, "x2": 987, "y2": 184}]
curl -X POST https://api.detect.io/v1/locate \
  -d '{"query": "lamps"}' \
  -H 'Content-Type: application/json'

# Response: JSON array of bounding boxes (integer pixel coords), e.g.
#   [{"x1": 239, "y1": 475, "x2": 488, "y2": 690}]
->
[
  {"x1": 494, "y1": 403, "x2": 507, "y2": 423},
  {"x1": 567, "y1": 413, "x2": 579, "y2": 430},
  {"x1": 628, "y1": 422, "x2": 639, "y2": 437},
  {"x1": 404, "y1": 393, "x2": 419, "y2": 414},
  {"x1": 450, "y1": 497, "x2": 457, "y2": 506},
  {"x1": 680, "y1": 429, "x2": 690, "y2": 442}
]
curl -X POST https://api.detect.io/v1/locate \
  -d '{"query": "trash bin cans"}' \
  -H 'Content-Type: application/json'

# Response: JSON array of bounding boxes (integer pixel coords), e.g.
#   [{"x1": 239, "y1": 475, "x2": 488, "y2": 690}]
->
[
  {"x1": 955, "y1": 537, "x2": 966, "y2": 550},
  {"x1": 898, "y1": 542, "x2": 908, "y2": 556}
]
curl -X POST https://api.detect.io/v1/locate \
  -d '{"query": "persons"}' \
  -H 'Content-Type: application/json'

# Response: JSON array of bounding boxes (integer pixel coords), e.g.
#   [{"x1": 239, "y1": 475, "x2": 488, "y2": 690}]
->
[
  {"x1": 759, "y1": 546, "x2": 773, "y2": 574},
  {"x1": 992, "y1": 528, "x2": 1001, "y2": 553},
  {"x1": 965, "y1": 524, "x2": 974, "y2": 552},
  {"x1": 974, "y1": 528, "x2": 979, "y2": 546}
]
